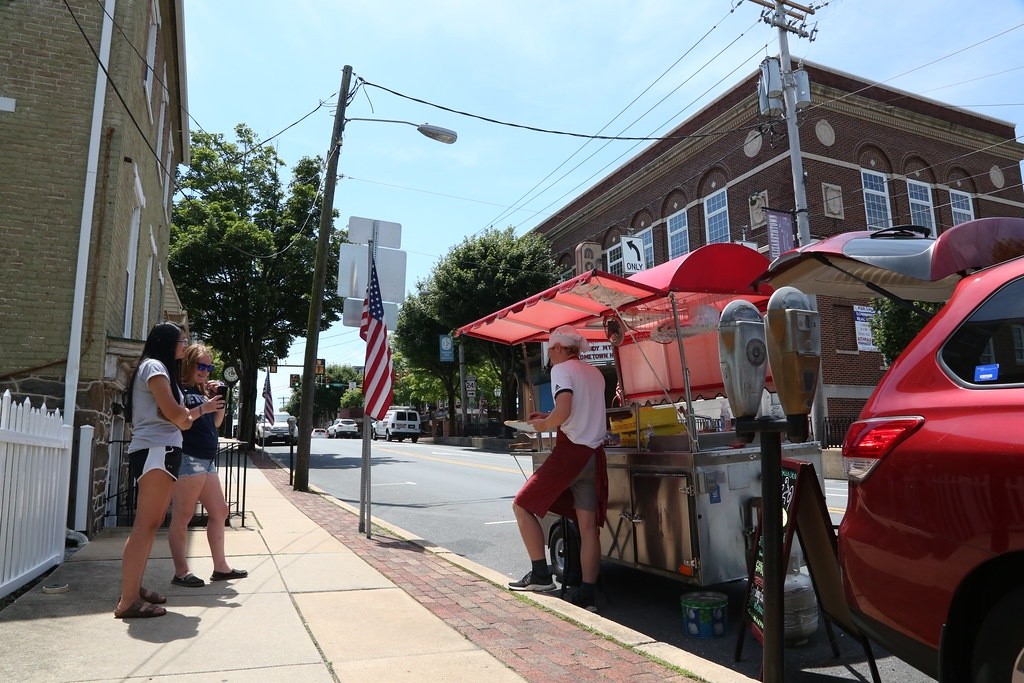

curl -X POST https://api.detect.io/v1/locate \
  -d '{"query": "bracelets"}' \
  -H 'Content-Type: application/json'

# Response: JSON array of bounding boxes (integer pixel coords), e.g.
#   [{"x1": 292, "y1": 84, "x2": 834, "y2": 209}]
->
[{"x1": 199, "y1": 404, "x2": 203, "y2": 416}]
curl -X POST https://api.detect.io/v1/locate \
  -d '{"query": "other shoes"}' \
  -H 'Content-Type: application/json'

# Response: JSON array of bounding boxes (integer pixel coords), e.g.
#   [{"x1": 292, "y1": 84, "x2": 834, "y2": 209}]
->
[
  {"x1": 170, "y1": 573, "x2": 205, "y2": 587},
  {"x1": 210, "y1": 569, "x2": 248, "y2": 580}
]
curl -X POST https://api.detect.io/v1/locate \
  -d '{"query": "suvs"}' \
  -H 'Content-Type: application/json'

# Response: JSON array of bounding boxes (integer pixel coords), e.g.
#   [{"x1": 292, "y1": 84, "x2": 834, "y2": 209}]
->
[
  {"x1": 371, "y1": 406, "x2": 421, "y2": 442},
  {"x1": 746, "y1": 214, "x2": 1024, "y2": 683},
  {"x1": 255, "y1": 412, "x2": 299, "y2": 446},
  {"x1": 327, "y1": 419, "x2": 358, "y2": 438}
]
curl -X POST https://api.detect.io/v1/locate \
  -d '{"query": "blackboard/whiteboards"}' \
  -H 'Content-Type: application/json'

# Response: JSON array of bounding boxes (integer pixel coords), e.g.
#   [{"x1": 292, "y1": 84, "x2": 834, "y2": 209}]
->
[{"x1": 743, "y1": 456, "x2": 870, "y2": 647}]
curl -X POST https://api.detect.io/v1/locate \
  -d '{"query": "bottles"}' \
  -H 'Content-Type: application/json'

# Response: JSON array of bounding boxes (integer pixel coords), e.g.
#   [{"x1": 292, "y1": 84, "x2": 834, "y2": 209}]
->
[{"x1": 720, "y1": 399, "x2": 731, "y2": 431}]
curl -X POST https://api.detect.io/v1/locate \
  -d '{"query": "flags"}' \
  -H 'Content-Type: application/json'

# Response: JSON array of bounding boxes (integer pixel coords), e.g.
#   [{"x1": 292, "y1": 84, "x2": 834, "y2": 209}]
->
[
  {"x1": 262, "y1": 366, "x2": 275, "y2": 426},
  {"x1": 360, "y1": 248, "x2": 395, "y2": 421}
]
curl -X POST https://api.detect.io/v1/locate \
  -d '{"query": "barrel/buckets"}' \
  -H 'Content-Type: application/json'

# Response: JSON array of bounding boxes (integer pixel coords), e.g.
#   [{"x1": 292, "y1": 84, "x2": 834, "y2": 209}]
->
[{"x1": 681, "y1": 591, "x2": 728, "y2": 640}]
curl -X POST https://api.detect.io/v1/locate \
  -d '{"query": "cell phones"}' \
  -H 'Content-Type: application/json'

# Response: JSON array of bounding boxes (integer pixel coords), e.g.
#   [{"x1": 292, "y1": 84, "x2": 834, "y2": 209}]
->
[{"x1": 217, "y1": 386, "x2": 228, "y2": 409}]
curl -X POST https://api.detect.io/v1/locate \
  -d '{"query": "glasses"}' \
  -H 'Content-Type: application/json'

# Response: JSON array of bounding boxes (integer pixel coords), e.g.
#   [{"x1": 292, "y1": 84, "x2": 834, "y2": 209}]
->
[
  {"x1": 178, "y1": 339, "x2": 189, "y2": 348},
  {"x1": 194, "y1": 361, "x2": 215, "y2": 372}
]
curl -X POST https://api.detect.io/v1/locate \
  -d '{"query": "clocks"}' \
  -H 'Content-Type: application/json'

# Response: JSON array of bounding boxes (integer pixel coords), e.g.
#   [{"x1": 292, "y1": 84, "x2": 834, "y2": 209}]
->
[{"x1": 221, "y1": 362, "x2": 240, "y2": 389}]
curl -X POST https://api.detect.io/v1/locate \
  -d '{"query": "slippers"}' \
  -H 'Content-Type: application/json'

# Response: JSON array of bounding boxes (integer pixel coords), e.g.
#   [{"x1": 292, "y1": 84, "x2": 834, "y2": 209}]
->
[
  {"x1": 118, "y1": 587, "x2": 167, "y2": 604},
  {"x1": 114, "y1": 598, "x2": 166, "y2": 618}
]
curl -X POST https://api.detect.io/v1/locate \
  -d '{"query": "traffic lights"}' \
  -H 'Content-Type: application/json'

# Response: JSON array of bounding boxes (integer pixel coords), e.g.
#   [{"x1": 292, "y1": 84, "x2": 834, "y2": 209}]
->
[
  {"x1": 295, "y1": 382, "x2": 300, "y2": 387},
  {"x1": 326, "y1": 377, "x2": 331, "y2": 388}
]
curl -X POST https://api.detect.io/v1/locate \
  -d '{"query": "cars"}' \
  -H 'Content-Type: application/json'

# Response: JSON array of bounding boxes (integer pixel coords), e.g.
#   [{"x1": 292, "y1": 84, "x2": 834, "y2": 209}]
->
[{"x1": 311, "y1": 428, "x2": 327, "y2": 438}]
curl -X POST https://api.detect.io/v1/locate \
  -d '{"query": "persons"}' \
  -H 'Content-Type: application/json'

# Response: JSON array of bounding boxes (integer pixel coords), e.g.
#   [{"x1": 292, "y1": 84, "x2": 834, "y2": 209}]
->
[
  {"x1": 167, "y1": 344, "x2": 248, "y2": 587},
  {"x1": 114, "y1": 321, "x2": 193, "y2": 618},
  {"x1": 507, "y1": 325, "x2": 609, "y2": 613}
]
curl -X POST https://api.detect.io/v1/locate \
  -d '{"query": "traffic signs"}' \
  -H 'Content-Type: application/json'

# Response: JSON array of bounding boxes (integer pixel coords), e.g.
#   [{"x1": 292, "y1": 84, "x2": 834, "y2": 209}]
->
[{"x1": 620, "y1": 235, "x2": 646, "y2": 274}]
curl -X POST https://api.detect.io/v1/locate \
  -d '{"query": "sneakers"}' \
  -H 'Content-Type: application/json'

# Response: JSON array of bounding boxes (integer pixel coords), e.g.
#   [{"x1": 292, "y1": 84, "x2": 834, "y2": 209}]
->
[
  {"x1": 508, "y1": 571, "x2": 556, "y2": 591},
  {"x1": 570, "y1": 591, "x2": 597, "y2": 613}
]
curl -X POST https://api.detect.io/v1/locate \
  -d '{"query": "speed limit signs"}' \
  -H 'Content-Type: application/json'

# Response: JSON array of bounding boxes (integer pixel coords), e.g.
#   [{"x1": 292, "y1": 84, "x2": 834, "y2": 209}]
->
[{"x1": 465, "y1": 374, "x2": 476, "y2": 391}]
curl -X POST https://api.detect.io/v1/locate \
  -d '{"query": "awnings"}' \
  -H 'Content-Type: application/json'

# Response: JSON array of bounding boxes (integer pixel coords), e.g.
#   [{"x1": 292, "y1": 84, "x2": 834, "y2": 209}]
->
[{"x1": 455, "y1": 265, "x2": 706, "y2": 453}]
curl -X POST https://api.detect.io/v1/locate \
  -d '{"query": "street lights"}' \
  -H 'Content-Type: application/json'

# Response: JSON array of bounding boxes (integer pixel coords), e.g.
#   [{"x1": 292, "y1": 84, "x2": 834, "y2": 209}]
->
[{"x1": 293, "y1": 118, "x2": 458, "y2": 491}]
